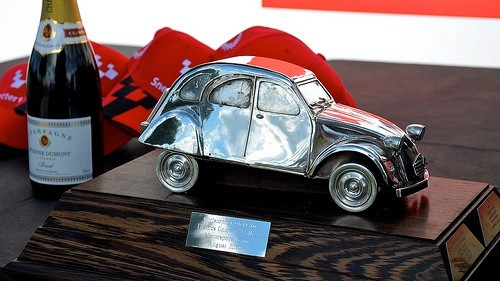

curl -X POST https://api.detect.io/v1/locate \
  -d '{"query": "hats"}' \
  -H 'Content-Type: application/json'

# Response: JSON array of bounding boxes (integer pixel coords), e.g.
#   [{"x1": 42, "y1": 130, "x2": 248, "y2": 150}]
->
[{"x1": 1, "y1": 25, "x2": 357, "y2": 158}]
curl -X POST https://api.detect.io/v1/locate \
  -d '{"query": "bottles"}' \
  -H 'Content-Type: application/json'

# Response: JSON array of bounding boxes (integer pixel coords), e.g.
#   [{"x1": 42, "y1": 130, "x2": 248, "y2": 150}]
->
[{"x1": 25, "y1": 0, "x2": 101, "y2": 201}]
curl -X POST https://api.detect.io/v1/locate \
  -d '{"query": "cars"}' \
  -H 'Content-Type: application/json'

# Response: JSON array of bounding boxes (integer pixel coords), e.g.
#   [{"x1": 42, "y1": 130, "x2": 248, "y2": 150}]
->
[{"x1": 135, "y1": 54, "x2": 430, "y2": 214}]
